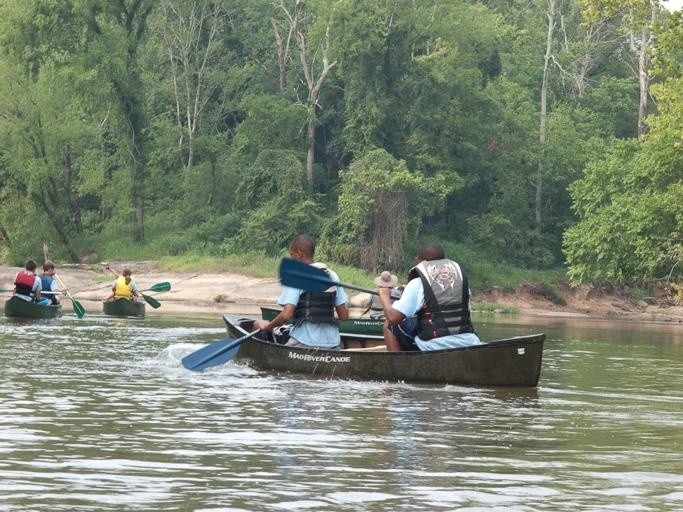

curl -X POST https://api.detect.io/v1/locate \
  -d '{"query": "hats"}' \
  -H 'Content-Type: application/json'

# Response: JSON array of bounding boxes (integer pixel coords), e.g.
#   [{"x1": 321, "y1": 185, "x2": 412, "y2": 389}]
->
[{"x1": 373, "y1": 270, "x2": 398, "y2": 288}]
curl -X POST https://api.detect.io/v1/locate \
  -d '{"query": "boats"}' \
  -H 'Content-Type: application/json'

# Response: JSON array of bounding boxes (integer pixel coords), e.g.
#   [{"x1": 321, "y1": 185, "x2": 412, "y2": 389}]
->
[
  {"x1": 102, "y1": 297, "x2": 146, "y2": 317},
  {"x1": 4, "y1": 295, "x2": 63, "y2": 319}
]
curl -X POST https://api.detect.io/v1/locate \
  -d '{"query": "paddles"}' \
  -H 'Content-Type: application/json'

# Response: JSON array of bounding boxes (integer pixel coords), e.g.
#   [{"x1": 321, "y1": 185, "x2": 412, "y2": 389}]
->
[
  {"x1": 107, "y1": 268, "x2": 161, "y2": 308},
  {"x1": 54, "y1": 273, "x2": 86, "y2": 318},
  {"x1": 0, "y1": 287, "x2": 67, "y2": 295},
  {"x1": 278, "y1": 257, "x2": 400, "y2": 300},
  {"x1": 181, "y1": 328, "x2": 262, "y2": 372},
  {"x1": 137, "y1": 282, "x2": 171, "y2": 292}
]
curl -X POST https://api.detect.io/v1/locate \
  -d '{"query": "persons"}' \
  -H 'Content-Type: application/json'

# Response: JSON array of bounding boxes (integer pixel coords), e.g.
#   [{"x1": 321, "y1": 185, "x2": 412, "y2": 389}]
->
[
  {"x1": 37, "y1": 260, "x2": 68, "y2": 305},
  {"x1": 347, "y1": 271, "x2": 402, "y2": 319},
  {"x1": 246, "y1": 235, "x2": 348, "y2": 350},
  {"x1": 111, "y1": 267, "x2": 141, "y2": 302},
  {"x1": 379, "y1": 243, "x2": 472, "y2": 351},
  {"x1": 12, "y1": 260, "x2": 52, "y2": 306}
]
